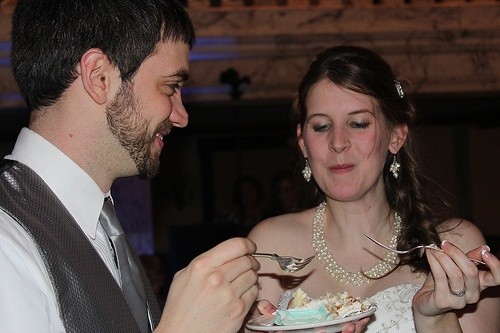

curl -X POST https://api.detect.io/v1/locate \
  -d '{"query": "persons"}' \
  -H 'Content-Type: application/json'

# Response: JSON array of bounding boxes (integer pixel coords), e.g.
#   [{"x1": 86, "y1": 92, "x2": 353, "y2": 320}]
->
[
  {"x1": 0, "y1": 0, "x2": 261, "y2": 333},
  {"x1": 234, "y1": 46, "x2": 500, "y2": 333}
]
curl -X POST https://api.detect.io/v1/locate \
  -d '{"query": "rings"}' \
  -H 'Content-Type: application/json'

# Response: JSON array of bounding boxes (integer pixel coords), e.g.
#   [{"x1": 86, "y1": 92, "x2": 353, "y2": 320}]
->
[{"x1": 450, "y1": 285, "x2": 467, "y2": 297}]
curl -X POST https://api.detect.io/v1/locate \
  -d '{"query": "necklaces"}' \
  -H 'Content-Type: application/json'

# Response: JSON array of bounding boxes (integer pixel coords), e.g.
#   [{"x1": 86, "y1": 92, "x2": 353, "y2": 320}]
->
[{"x1": 311, "y1": 201, "x2": 403, "y2": 286}]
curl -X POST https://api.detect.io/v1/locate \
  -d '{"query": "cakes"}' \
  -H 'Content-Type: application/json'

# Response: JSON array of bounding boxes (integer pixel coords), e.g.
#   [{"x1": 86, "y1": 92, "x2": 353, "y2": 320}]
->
[{"x1": 271, "y1": 288, "x2": 377, "y2": 324}]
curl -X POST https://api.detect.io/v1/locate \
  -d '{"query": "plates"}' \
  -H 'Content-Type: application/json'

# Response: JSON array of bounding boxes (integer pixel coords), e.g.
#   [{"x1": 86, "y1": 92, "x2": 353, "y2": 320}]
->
[{"x1": 245, "y1": 308, "x2": 376, "y2": 333}]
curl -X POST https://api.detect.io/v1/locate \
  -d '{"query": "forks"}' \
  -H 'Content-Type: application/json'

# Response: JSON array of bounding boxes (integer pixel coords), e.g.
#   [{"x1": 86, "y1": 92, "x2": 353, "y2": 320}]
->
[
  {"x1": 252, "y1": 252, "x2": 315, "y2": 273},
  {"x1": 363, "y1": 233, "x2": 488, "y2": 266}
]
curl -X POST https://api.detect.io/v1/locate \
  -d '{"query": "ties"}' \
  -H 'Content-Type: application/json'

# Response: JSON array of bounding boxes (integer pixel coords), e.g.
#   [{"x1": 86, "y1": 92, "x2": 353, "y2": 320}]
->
[{"x1": 99, "y1": 196, "x2": 152, "y2": 333}]
